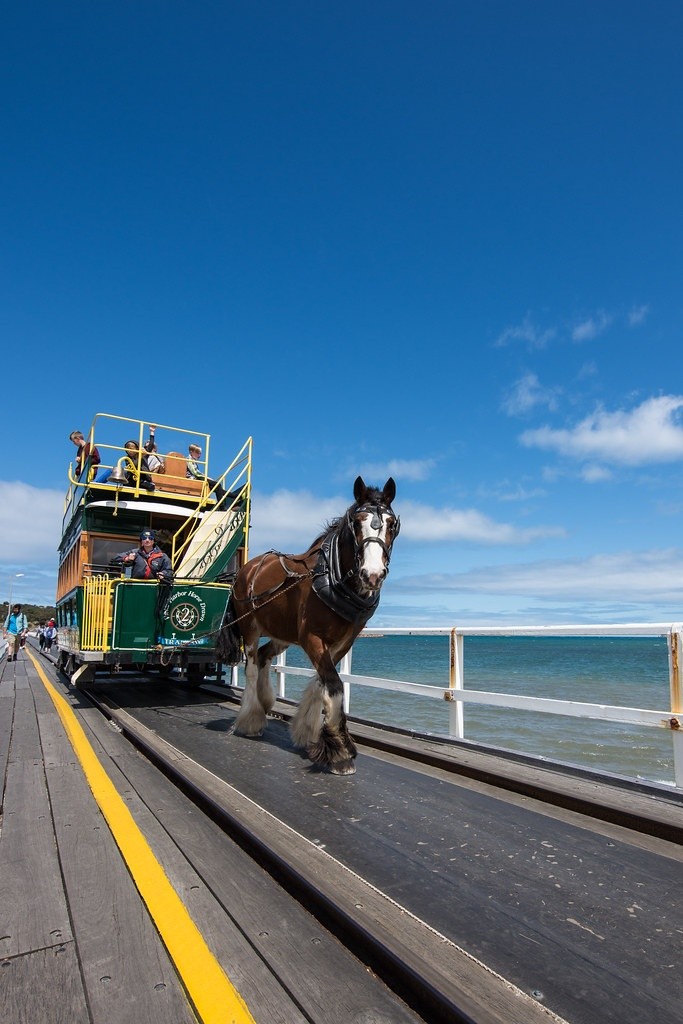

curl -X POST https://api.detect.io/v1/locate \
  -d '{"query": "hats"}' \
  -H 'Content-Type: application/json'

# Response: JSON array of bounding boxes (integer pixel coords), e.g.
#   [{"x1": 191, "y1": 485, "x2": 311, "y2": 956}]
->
[
  {"x1": 51, "y1": 618, "x2": 54, "y2": 620},
  {"x1": 140, "y1": 529, "x2": 155, "y2": 536},
  {"x1": 48, "y1": 622, "x2": 54, "y2": 627}
]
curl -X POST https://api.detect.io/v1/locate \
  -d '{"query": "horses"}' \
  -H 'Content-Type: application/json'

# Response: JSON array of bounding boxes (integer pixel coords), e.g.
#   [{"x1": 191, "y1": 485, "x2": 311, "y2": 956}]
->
[{"x1": 215, "y1": 476, "x2": 400, "y2": 776}]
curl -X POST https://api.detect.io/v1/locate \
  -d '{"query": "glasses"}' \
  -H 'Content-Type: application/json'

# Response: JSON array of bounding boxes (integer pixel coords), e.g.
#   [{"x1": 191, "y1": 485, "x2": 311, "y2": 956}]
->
[
  {"x1": 141, "y1": 537, "x2": 154, "y2": 540},
  {"x1": 144, "y1": 445, "x2": 149, "y2": 448}
]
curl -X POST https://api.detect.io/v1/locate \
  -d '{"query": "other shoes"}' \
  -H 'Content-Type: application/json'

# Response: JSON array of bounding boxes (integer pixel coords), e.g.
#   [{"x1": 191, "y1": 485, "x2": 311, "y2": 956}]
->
[
  {"x1": 7, "y1": 655, "x2": 12, "y2": 662},
  {"x1": 13, "y1": 654, "x2": 17, "y2": 661}
]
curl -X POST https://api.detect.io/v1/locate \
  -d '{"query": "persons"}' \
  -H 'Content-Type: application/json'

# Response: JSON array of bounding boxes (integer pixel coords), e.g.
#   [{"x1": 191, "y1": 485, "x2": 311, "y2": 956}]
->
[
  {"x1": 124, "y1": 425, "x2": 156, "y2": 492},
  {"x1": 35, "y1": 617, "x2": 57, "y2": 654},
  {"x1": 3, "y1": 604, "x2": 28, "y2": 663},
  {"x1": 186, "y1": 444, "x2": 247, "y2": 511},
  {"x1": 111, "y1": 529, "x2": 172, "y2": 579},
  {"x1": 69, "y1": 430, "x2": 101, "y2": 482},
  {"x1": 145, "y1": 440, "x2": 166, "y2": 474}
]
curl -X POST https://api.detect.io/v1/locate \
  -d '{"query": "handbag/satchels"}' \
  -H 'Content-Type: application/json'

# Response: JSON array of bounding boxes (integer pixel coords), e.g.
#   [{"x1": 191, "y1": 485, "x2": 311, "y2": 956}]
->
[{"x1": 20, "y1": 637, "x2": 26, "y2": 646}]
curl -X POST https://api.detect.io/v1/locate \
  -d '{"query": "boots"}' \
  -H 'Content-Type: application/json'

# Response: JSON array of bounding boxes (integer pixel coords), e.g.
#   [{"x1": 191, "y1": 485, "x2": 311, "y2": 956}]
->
[
  {"x1": 48, "y1": 648, "x2": 50, "y2": 654},
  {"x1": 44, "y1": 648, "x2": 47, "y2": 653}
]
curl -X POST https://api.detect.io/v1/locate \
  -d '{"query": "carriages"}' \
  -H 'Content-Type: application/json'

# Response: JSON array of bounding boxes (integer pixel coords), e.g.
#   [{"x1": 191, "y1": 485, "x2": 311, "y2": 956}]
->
[{"x1": 50, "y1": 412, "x2": 401, "y2": 776}]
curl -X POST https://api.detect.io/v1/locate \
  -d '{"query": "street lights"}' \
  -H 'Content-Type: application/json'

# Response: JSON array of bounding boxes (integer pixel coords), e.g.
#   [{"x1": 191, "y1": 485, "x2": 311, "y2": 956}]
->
[{"x1": 8, "y1": 573, "x2": 25, "y2": 616}]
